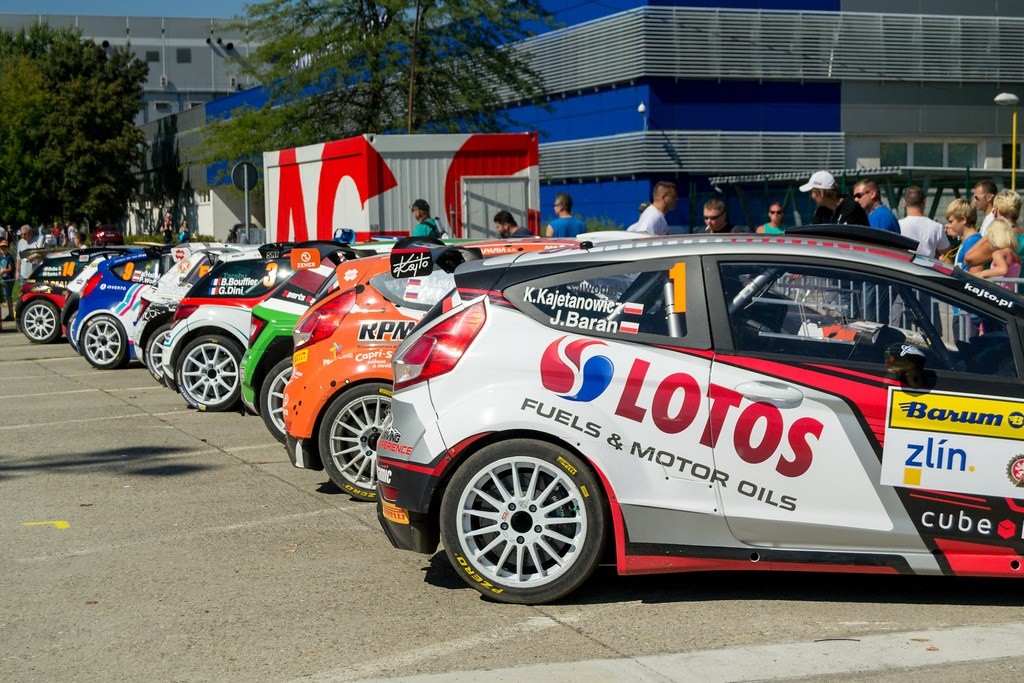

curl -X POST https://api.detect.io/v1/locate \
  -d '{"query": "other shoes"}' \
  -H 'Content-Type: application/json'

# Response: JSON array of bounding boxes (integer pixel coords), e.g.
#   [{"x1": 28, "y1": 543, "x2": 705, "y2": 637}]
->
[{"x1": 2, "y1": 315, "x2": 14, "y2": 321}]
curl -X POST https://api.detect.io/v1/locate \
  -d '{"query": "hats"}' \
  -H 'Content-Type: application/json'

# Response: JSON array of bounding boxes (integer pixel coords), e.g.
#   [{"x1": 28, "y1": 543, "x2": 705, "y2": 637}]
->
[
  {"x1": 799, "y1": 171, "x2": 836, "y2": 192},
  {"x1": 0, "y1": 240, "x2": 8, "y2": 247},
  {"x1": 412, "y1": 199, "x2": 429, "y2": 211}
]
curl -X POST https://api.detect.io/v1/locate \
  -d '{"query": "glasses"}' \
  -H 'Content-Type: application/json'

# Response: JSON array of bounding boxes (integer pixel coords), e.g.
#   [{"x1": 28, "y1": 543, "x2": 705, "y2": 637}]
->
[
  {"x1": 703, "y1": 213, "x2": 724, "y2": 219},
  {"x1": 975, "y1": 194, "x2": 995, "y2": 201},
  {"x1": 769, "y1": 211, "x2": 783, "y2": 214},
  {"x1": 854, "y1": 191, "x2": 870, "y2": 198}
]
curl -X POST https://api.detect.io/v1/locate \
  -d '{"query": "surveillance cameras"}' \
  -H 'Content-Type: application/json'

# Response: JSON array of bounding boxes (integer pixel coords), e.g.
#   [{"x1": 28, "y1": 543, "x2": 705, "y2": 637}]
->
[{"x1": 638, "y1": 104, "x2": 646, "y2": 115}]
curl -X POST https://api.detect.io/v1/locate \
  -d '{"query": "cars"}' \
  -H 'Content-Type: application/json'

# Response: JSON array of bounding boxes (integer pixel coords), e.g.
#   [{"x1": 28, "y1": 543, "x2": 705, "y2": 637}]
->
[
  {"x1": 369, "y1": 222, "x2": 1024, "y2": 612},
  {"x1": 12, "y1": 237, "x2": 366, "y2": 446},
  {"x1": 91, "y1": 224, "x2": 124, "y2": 243},
  {"x1": 284, "y1": 236, "x2": 822, "y2": 503}
]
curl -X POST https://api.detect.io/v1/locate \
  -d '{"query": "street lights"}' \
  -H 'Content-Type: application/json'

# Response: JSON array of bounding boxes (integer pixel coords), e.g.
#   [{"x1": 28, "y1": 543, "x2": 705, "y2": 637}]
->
[{"x1": 993, "y1": 93, "x2": 1017, "y2": 194}]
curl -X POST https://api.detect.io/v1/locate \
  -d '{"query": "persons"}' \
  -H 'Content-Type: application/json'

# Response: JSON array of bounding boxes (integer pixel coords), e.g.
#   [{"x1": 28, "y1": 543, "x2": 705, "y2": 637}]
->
[
  {"x1": 179, "y1": 221, "x2": 190, "y2": 243},
  {"x1": 797, "y1": 169, "x2": 886, "y2": 331},
  {"x1": 160, "y1": 211, "x2": 173, "y2": 244},
  {"x1": 888, "y1": 185, "x2": 951, "y2": 329},
  {"x1": 947, "y1": 181, "x2": 1024, "y2": 337},
  {"x1": 756, "y1": 202, "x2": 786, "y2": 236},
  {"x1": 627, "y1": 181, "x2": 679, "y2": 237},
  {"x1": 408, "y1": 199, "x2": 444, "y2": 237},
  {"x1": 878, "y1": 344, "x2": 937, "y2": 397},
  {"x1": 0, "y1": 221, "x2": 89, "y2": 332},
  {"x1": 854, "y1": 179, "x2": 902, "y2": 322},
  {"x1": 545, "y1": 193, "x2": 587, "y2": 237},
  {"x1": 692, "y1": 200, "x2": 751, "y2": 233},
  {"x1": 494, "y1": 211, "x2": 533, "y2": 239}
]
